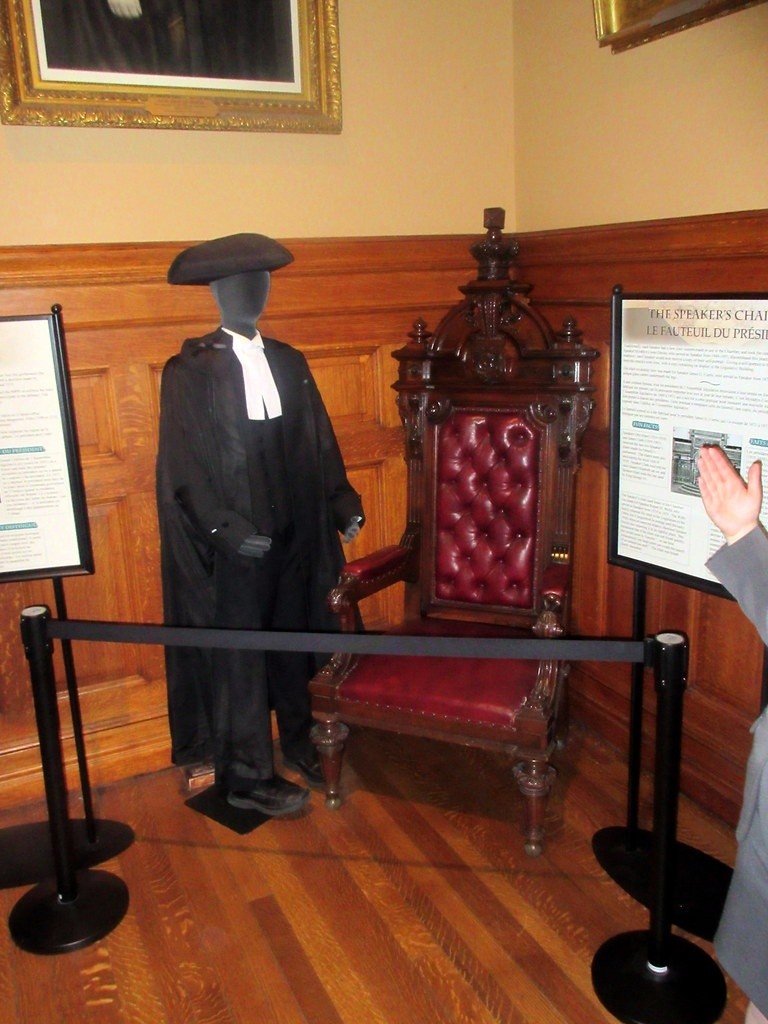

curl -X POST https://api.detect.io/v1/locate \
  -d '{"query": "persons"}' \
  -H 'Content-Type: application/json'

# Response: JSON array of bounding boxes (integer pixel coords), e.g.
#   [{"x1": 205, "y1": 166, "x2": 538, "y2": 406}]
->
[
  {"x1": 696, "y1": 447, "x2": 768, "y2": 1024},
  {"x1": 155, "y1": 233, "x2": 366, "y2": 816}
]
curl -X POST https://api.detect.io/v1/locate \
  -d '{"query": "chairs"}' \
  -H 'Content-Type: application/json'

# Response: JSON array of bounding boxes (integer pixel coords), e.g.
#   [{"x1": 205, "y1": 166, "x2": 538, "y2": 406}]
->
[{"x1": 305, "y1": 204, "x2": 599, "y2": 854}]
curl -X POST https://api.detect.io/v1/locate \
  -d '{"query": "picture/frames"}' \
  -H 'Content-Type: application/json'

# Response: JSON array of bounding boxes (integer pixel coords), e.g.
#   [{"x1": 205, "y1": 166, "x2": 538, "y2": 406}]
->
[
  {"x1": 591, "y1": 0, "x2": 768, "y2": 42},
  {"x1": 0, "y1": 0, "x2": 345, "y2": 135}
]
